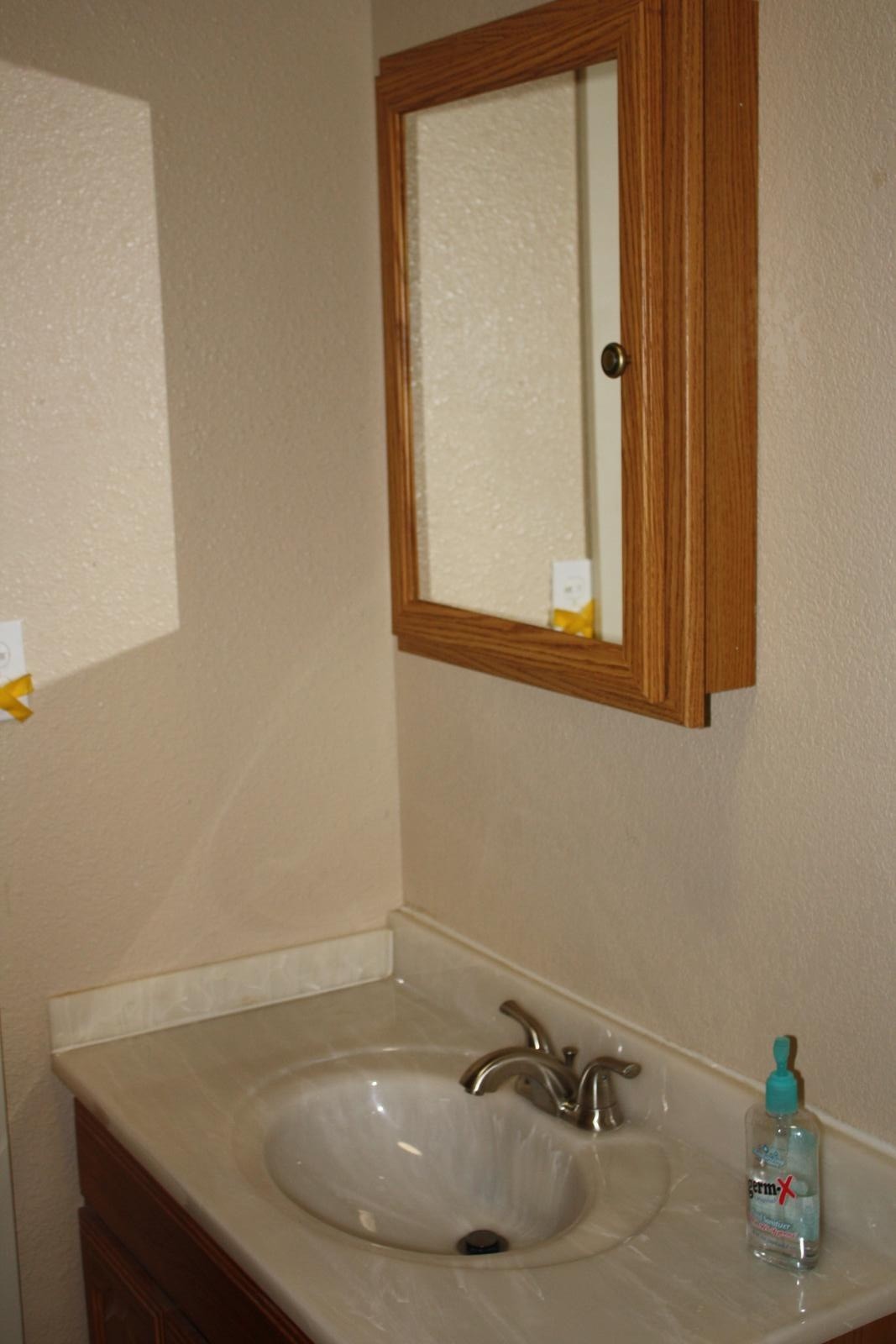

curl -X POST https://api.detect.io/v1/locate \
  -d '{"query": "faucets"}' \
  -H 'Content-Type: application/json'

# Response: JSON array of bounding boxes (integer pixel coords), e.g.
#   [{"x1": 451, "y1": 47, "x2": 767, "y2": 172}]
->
[{"x1": 457, "y1": 996, "x2": 647, "y2": 1133}]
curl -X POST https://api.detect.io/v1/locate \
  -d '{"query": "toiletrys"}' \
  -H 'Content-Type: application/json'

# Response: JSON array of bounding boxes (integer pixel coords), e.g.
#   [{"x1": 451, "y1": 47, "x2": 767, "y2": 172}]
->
[{"x1": 747, "y1": 1035, "x2": 820, "y2": 1273}]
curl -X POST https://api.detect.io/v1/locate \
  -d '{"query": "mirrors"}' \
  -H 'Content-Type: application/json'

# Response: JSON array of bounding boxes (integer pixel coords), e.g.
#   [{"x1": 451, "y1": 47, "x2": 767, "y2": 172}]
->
[{"x1": 399, "y1": 54, "x2": 624, "y2": 644}]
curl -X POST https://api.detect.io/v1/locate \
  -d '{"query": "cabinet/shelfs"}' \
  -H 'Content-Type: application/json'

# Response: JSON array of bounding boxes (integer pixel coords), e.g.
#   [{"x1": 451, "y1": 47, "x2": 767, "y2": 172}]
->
[
  {"x1": 374, "y1": 0, "x2": 761, "y2": 730},
  {"x1": 74, "y1": 1097, "x2": 310, "y2": 1343}
]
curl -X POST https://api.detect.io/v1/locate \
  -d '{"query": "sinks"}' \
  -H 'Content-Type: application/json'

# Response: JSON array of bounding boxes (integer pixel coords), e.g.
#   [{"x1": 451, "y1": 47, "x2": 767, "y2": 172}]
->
[{"x1": 226, "y1": 1040, "x2": 673, "y2": 1275}]
